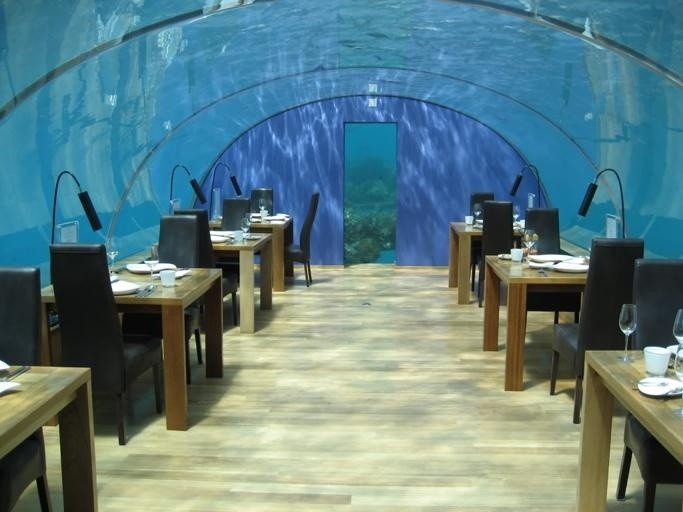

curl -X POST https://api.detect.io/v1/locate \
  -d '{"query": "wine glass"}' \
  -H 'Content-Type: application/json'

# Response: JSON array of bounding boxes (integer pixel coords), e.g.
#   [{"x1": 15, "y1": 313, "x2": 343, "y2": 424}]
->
[
  {"x1": 673, "y1": 309, "x2": 683, "y2": 346},
  {"x1": 513, "y1": 205, "x2": 519, "y2": 222},
  {"x1": 673, "y1": 348, "x2": 683, "y2": 417},
  {"x1": 474, "y1": 203, "x2": 481, "y2": 224},
  {"x1": 258, "y1": 199, "x2": 267, "y2": 211},
  {"x1": 104, "y1": 238, "x2": 120, "y2": 275},
  {"x1": 522, "y1": 229, "x2": 535, "y2": 262},
  {"x1": 619, "y1": 303, "x2": 637, "y2": 362},
  {"x1": 144, "y1": 245, "x2": 159, "y2": 283},
  {"x1": 240, "y1": 218, "x2": 250, "y2": 235}
]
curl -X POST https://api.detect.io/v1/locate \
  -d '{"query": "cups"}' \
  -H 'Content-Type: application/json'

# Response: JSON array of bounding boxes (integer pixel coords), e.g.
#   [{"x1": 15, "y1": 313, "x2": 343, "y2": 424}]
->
[
  {"x1": 235, "y1": 231, "x2": 243, "y2": 242},
  {"x1": 511, "y1": 249, "x2": 522, "y2": 262},
  {"x1": 644, "y1": 346, "x2": 671, "y2": 378},
  {"x1": 465, "y1": 216, "x2": 473, "y2": 225}
]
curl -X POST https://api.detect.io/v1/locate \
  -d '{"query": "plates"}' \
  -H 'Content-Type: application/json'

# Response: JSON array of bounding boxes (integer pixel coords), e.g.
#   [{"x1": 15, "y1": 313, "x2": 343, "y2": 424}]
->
[
  {"x1": 109, "y1": 263, "x2": 191, "y2": 295},
  {"x1": 244, "y1": 236, "x2": 261, "y2": 240},
  {"x1": 528, "y1": 254, "x2": 589, "y2": 273},
  {"x1": 250, "y1": 212, "x2": 289, "y2": 223},
  {"x1": 475, "y1": 220, "x2": 483, "y2": 224},
  {"x1": 210, "y1": 231, "x2": 235, "y2": 236},
  {"x1": 498, "y1": 254, "x2": 511, "y2": 260},
  {"x1": 0, "y1": 382, "x2": 20, "y2": 394},
  {"x1": 667, "y1": 344, "x2": 680, "y2": 353},
  {"x1": 0, "y1": 360, "x2": 10, "y2": 371},
  {"x1": 211, "y1": 235, "x2": 230, "y2": 243},
  {"x1": 638, "y1": 377, "x2": 683, "y2": 397}
]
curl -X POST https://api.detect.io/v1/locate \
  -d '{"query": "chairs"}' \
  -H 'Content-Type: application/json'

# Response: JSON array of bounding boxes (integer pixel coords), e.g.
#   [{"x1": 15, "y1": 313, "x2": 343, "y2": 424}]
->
[
  {"x1": 173, "y1": 209, "x2": 238, "y2": 327},
  {"x1": 287, "y1": 192, "x2": 321, "y2": 288},
  {"x1": 478, "y1": 199, "x2": 513, "y2": 307},
  {"x1": 49, "y1": 243, "x2": 165, "y2": 445},
  {"x1": 250, "y1": 188, "x2": 272, "y2": 216},
  {"x1": 470, "y1": 192, "x2": 494, "y2": 291},
  {"x1": 123, "y1": 215, "x2": 203, "y2": 386},
  {"x1": 543, "y1": 239, "x2": 644, "y2": 422},
  {"x1": 222, "y1": 198, "x2": 252, "y2": 235},
  {"x1": 524, "y1": 207, "x2": 581, "y2": 325},
  {"x1": 0, "y1": 267, "x2": 52, "y2": 512},
  {"x1": 616, "y1": 259, "x2": 682, "y2": 512}
]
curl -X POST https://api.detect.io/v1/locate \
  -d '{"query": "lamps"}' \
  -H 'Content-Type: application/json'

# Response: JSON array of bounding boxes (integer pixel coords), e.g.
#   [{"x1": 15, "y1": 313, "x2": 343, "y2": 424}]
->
[
  {"x1": 578, "y1": 170, "x2": 625, "y2": 241},
  {"x1": 169, "y1": 164, "x2": 207, "y2": 214},
  {"x1": 510, "y1": 165, "x2": 540, "y2": 208},
  {"x1": 51, "y1": 172, "x2": 102, "y2": 244},
  {"x1": 209, "y1": 162, "x2": 242, "y2": 219}
]
